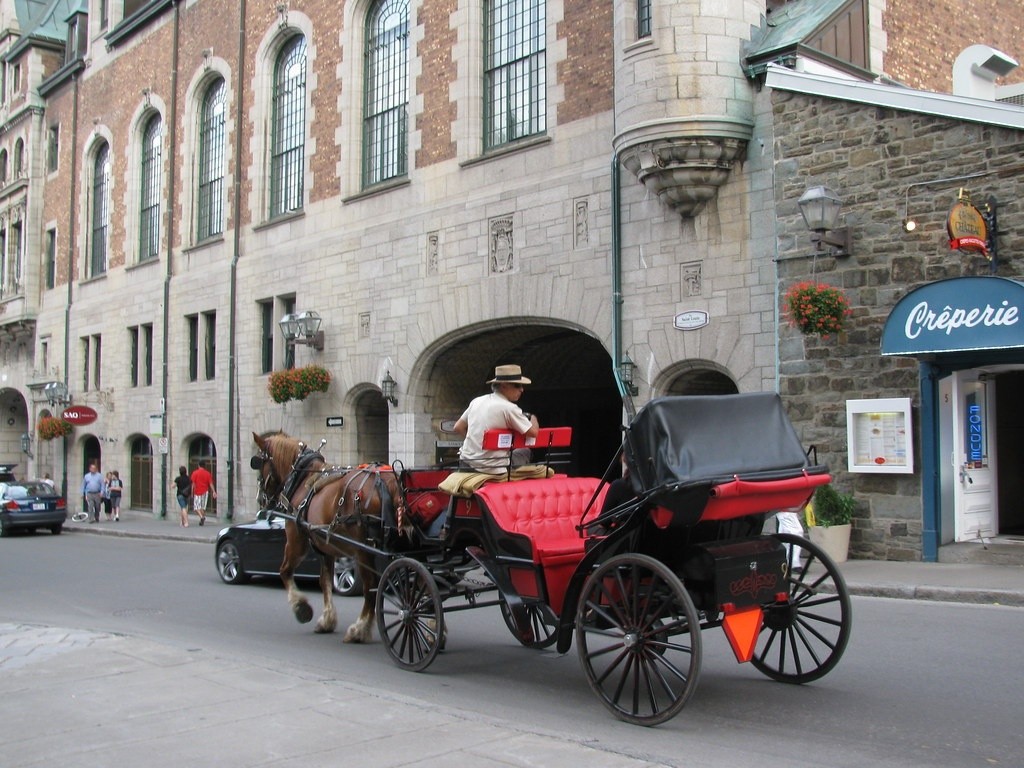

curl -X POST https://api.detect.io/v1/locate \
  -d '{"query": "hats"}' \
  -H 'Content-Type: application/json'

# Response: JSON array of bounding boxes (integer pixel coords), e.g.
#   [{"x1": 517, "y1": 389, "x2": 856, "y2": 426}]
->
[{"x1": 487, "y1": 365, "x2": 531, "y2": 384}]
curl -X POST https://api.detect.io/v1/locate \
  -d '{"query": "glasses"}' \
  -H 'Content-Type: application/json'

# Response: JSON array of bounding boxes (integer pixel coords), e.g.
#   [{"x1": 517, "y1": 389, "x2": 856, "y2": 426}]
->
[{"x1": 507, "y1": 383, "x2": 521, "y2": 389}]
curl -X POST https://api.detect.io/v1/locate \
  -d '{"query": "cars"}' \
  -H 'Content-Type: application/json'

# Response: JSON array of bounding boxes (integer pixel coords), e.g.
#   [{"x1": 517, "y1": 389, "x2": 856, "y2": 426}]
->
[
  {"x1": 0, "y1": 481, "x2": 67, "y2": 537},
  {"x1": 214, "y1": 507, "x2": 469, "y2": 597}
]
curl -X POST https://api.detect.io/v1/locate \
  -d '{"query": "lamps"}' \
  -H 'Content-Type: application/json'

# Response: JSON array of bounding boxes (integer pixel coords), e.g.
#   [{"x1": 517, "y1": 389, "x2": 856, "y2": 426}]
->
[
  {"x1": 795, "y1": 185, "x2": 854, "y2": 258},
  {"x1": 44, "y1": 382, "x2": 73, "y2": 408},
  {"x1": 381, "y1": 370, "x2": 399, "y2": 407},
  {"x1": 278, "y1": 310, "x2": 324, "y2": 351},
  {"x1": 20, "y1": 434, "x2": 34, "y2": 460}
]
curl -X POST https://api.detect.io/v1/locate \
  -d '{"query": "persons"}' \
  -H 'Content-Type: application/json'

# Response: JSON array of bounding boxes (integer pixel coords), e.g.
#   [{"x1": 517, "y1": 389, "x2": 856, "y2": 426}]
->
[
  {"x1": 102, "y1": 472, "x2": 113, "y2": 520},
  {"x1": 452, "y1": 364, "x2": 539, "y2": 476},
  {"x1": 42, "y1": 473, "x2": 54, "y2": 490},
  {"x1": 171, "y1": 466, "x2": 192, "y2": 528},
  {"x1": 191, "y1": 461, "x2": 217, "y2": 526},
  {"x1": 81, "y1": 464, "x2": 106, "y2": 522},
  {"x1": 107, "y1": 470, "x2": 123, "y2": 521}
]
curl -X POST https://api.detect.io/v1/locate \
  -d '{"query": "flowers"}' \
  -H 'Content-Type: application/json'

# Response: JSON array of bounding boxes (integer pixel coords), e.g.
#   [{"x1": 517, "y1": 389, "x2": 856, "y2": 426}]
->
[
  {"x1": 292, "y1": 364, "x2": 331, "y2": 402},
  {"x1": 266, "y1": 367, "x2": 304, "y2": 403},
  {"x1": 784, "y1": 280, "x2": 854, "y2": 339},
  {"x1": 36, "y1": 416, "x2": 73, "y2": 441}
]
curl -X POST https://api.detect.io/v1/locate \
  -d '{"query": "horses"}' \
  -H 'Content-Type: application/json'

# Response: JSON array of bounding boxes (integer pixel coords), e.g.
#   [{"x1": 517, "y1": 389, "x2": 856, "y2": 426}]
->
[{"x1": 251, "y1": 428, "x2": 449, "y2": 655}]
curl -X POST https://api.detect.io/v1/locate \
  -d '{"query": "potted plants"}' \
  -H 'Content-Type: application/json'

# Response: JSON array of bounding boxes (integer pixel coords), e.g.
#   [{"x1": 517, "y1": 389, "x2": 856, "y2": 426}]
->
[{"x1": 806, "y1": 483, "x2": 859, "y2": 564}]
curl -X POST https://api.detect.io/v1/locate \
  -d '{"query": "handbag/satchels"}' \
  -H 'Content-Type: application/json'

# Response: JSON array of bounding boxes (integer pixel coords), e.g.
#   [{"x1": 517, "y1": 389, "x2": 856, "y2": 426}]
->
[{"x1": 182, "y1": 485, "x2": 192, "y2": 499}]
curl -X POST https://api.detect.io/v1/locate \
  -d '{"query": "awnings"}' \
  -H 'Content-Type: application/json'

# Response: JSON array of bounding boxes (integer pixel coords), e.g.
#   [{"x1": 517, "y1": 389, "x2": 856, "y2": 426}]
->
[{"x1": 880, "y1": 275, "x2": 1024, "y2": 355}]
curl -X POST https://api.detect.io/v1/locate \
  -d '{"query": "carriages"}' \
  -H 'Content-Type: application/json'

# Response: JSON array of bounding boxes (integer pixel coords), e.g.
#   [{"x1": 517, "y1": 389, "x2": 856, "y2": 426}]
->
[{"x1": 249, "y1": 393, "x2": 852, "y2": 727}]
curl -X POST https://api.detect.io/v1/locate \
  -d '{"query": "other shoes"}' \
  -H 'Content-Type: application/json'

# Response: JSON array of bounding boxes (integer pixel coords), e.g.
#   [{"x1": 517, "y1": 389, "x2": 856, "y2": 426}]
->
[
  {"x1": 199, "y1": 517, "x2": 205, "y2": 525},
  {"x1": 115, "y1": 515, "x2": 119, "y2": 520}
]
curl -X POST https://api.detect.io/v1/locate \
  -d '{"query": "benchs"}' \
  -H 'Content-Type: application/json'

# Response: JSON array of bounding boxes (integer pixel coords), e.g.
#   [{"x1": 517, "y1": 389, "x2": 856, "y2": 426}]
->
[
  {"x1": 477, "y1": 476, "x2": 612, "y2": 616},
  {"x1": 405, "y1": 470, "x2": 453, "y2": 525},
  {"x1": 582, "y1": 510, "x2": 765, "y2": 573},
  {"x1": 454, "y1": 427, "x2": 573, "y2": 518}
]
